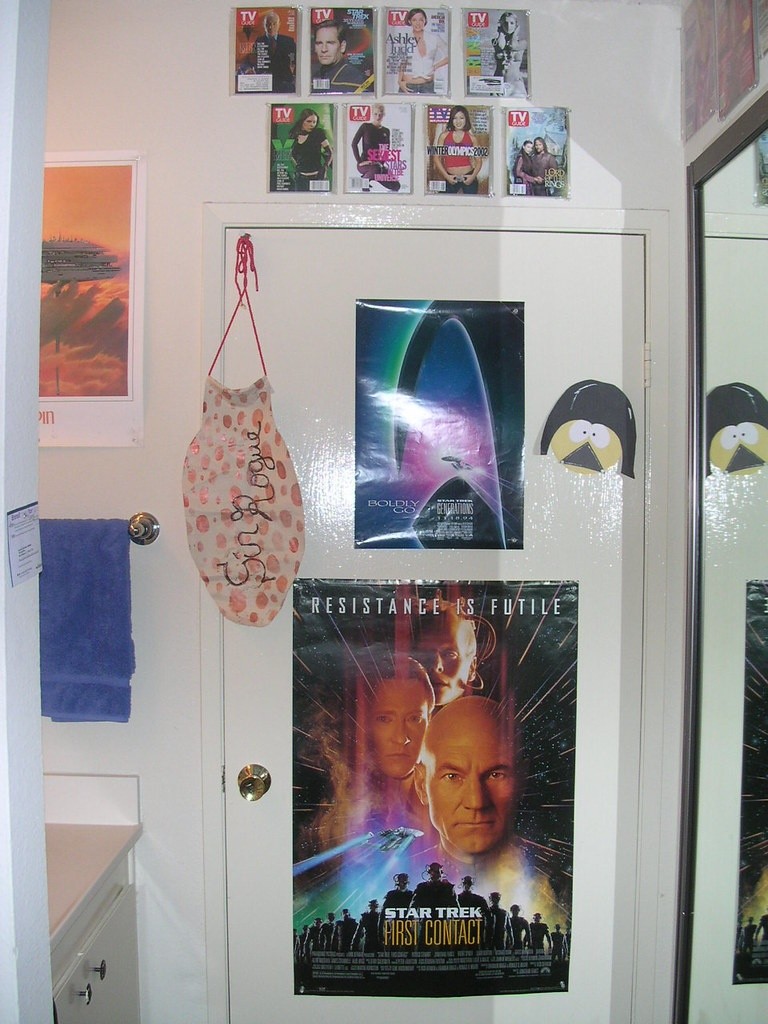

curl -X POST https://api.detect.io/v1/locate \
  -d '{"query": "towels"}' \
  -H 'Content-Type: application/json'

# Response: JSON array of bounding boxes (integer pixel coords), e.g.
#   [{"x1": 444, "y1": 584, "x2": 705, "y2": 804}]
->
[{"x1": 37, "y1": 518, "x2": 137, "y2": 724}]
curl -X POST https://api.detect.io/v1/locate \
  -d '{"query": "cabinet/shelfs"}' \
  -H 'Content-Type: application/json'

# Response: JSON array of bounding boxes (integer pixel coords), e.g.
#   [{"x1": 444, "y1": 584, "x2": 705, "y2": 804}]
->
[{"x1": 45, "y1": 822, "x2": 143, "y2": 1024}]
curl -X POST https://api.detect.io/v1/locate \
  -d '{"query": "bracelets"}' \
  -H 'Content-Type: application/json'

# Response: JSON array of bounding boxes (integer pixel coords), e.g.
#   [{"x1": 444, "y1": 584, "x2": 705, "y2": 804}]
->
[{"x1": 324, "y1": 163, "x2": 329, "y2": 168}]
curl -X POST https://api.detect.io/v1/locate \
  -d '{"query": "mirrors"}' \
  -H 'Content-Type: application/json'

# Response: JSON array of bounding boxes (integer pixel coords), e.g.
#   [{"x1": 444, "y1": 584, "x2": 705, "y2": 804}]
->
[{"x1": 670, "y1": 90, "x2": 768, "y2": 1024}]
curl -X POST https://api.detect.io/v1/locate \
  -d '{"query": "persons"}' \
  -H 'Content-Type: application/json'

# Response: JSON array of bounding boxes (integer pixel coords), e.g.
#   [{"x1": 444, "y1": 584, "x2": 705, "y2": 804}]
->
[
  {"x1": 313, "y1": 640, "x2": 437, "y2": 852},
  {"x1": 511, "y1": 137, "x2": 560, "y2": 195},
  {"x1": 288, "y1": 110, "x2": 333, "y2": 191},
  {"x1": 294, "y1": 862, "x2": 572, "y2": 965},
  {"x1": 392, "y1": 598, "x2": 479, "y2": 720},
  {"x1": 736, "y1": 914, "x2": 768, "y2": 958},
  {"x1": 311, "y1": 19, "x2": 374, "y2": 93},
  {"x1": 490, "y1": 11, "x2": 527, "y2": 96},
  {"x1": 413, "y1": 697, "x2": 519, "y2": 884},
  {"x1": 434, "y1": 105, "x2": 483, "y2": 194},
  {"x1": 398, "y1": 9, "x2": 448, "y2": 93},
  {"x1": 351, "y1": 105, "x2": 402, "y2": 190},
  {"x1": 238, "y1": 10, "x2": 296, "y2": 93}
]
